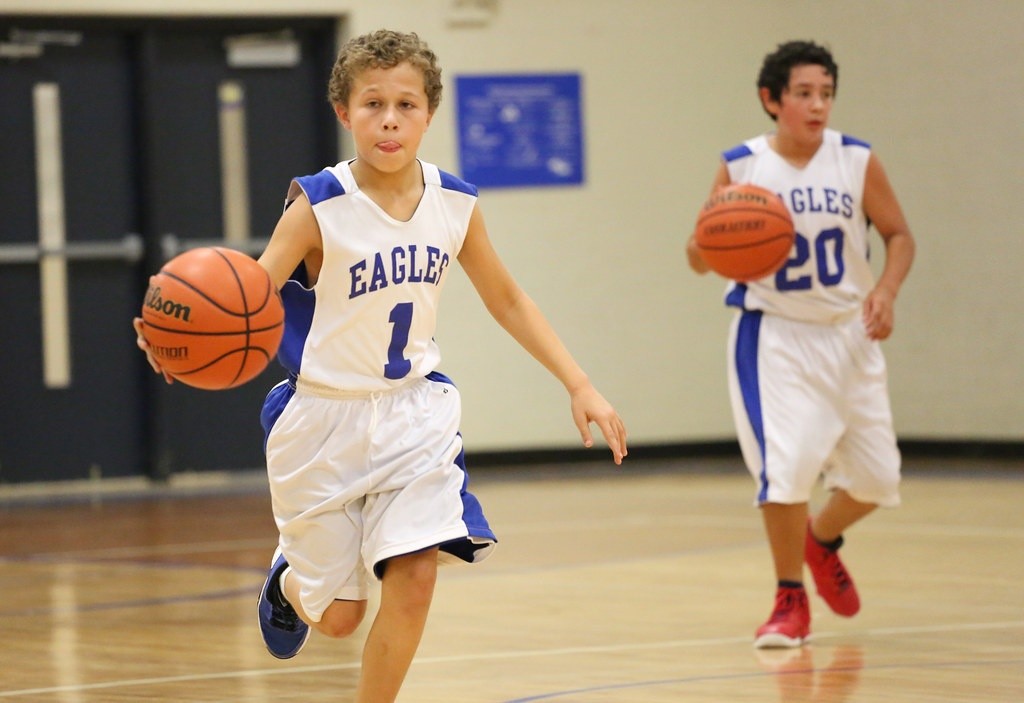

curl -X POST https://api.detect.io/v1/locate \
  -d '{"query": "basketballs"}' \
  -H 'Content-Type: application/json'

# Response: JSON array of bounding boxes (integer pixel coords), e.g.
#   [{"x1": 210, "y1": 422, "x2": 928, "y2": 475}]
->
[
  {"x1": 692, "y1": 183, "x2": 795, "y2": 286},
  {"x1": 139, "y1": 244, "x2": 287, "y2": 394}
]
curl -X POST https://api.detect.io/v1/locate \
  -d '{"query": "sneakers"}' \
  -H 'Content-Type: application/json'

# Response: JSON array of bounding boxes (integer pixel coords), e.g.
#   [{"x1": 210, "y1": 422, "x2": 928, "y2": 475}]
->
[
  {"x1": 754, "y1": 585, "x2": 812, "y2": 649},
  {"x1": 256, "y1": 544, "x2": 312, "y2": 660},
  {"x1": 803, "y1": 517, "x2": 860, "y2": 616}
]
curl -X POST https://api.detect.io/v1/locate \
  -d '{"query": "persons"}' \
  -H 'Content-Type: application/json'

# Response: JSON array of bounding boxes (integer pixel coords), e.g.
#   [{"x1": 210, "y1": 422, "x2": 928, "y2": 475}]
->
[
  {"x1": 133, "y1": 29, "x2": 629, "y2": 703},
  {"x1": 685, "y1": 38, "x2": 916, "y2": 651}
]
